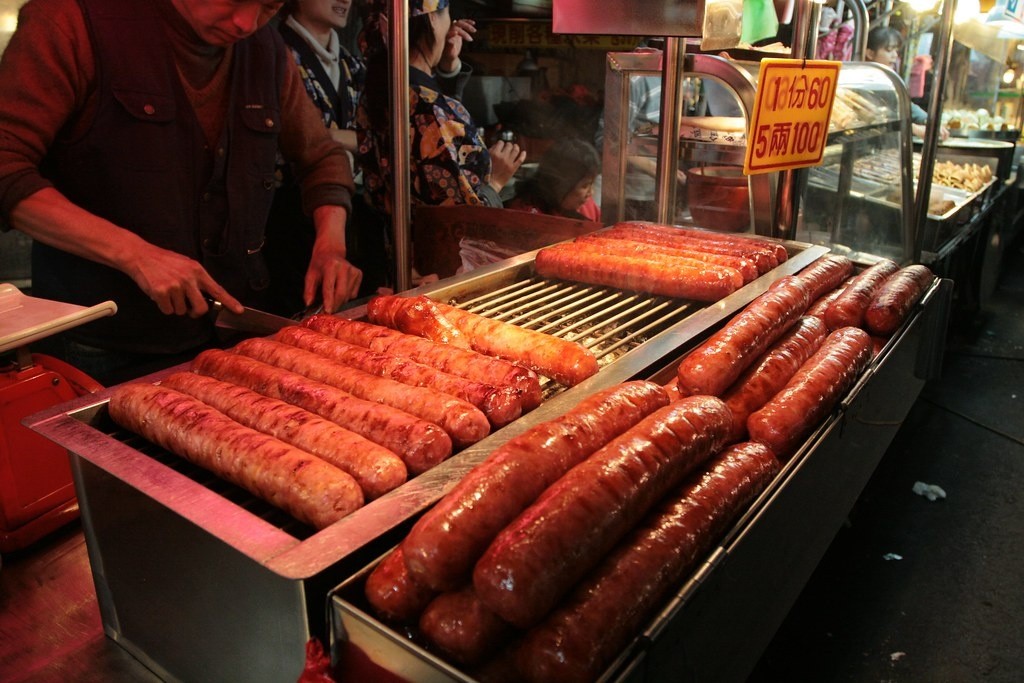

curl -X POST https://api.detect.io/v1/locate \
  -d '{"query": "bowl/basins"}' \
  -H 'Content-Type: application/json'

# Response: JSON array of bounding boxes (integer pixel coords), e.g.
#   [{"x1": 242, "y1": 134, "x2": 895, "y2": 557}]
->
[{"x1": 686, "y1": 166, "x2": 750, "y2": 232}]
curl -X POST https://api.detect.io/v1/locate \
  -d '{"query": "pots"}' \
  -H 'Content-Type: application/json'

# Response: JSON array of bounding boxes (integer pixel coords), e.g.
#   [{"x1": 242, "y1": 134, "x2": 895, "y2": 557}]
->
[
  {"x1": 947, "y1": 128, "x2": 1021, "y2": 143},
  {"x1": 913, "y1": 136, "x2": 1015, "y2": 180}
]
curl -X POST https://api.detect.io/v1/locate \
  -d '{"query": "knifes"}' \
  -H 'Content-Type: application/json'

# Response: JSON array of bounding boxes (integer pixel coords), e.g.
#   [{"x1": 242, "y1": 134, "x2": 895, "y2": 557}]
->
[{"x1": 186, "y1": 295, "x2": 301, "y2": 333}]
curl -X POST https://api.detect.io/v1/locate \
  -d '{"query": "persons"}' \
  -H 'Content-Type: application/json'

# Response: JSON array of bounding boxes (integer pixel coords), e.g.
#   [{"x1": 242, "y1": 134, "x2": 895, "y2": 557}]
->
[
  {"x1": 511, "y1": 139, "x2": 599, "y2": 225},
  {"x1": 864, "y1": 26, "x2": 950, "y2": 143},
  {"x1": 0, "y1": 0, "x2": 527, "y2": 379},
  {"x1": 594, "y1": 72, "x2": 687, "y2": 224}
]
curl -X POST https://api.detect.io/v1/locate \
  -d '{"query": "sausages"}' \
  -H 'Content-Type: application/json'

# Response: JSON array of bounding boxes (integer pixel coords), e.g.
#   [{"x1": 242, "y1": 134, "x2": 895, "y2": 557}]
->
[
  {"x1": 108, "y1": 298, "x2": 600, "y2": 530},
  {"x1": 534, "y1": 222, "x2": 788, "y2": 304},
  {"x1": 365, "y1": 254, "x2": 933, "y2": 683}
]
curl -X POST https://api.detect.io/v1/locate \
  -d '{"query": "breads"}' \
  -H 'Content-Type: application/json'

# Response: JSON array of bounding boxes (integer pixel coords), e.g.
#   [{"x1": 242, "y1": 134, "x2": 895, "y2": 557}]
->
[
  {"x1": 886, "y1": 159, "x2": 993, "y2": 215},
  {"x1": 832, "y1": 87, "x2": 881, "y2": 130}
]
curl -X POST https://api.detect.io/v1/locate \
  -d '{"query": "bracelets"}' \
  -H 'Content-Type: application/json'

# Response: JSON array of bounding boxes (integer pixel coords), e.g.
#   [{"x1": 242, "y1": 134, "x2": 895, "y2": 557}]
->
[{"x1": 436, "y1": 59, "x2": 461, "y2": 78}]
